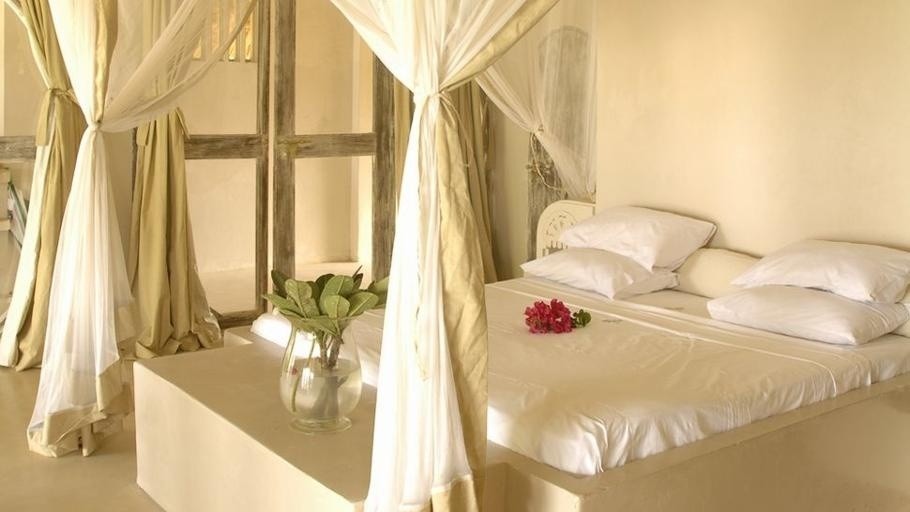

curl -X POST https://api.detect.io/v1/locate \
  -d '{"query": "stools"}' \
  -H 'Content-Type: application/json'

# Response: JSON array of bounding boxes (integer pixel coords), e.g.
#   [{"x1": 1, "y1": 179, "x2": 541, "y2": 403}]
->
[{"x1": 132, "y1": 339, "x2": 508, "y2": 512}]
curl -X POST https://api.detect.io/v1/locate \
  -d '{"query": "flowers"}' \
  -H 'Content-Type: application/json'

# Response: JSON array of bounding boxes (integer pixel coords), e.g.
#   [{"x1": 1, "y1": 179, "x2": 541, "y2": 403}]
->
[{"x1": 524, "y1": 297, "x2": 592, "y2": 335}]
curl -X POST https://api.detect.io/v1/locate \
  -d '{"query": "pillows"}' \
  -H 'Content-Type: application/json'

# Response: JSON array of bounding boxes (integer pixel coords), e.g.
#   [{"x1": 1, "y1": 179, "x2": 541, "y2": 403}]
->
[
  {"x1": 557, "y1": 205, "x2": 718, "y2": 273},
  {"x1": 519, "y1": 247, "x2": 681, "y2": 303},
  {"x1": 729, "y1": 238, "x2": 910, "y2": 304},
  {"x1": 707, "y1": 286, "x2": 910, "y2": 345},
  {"x1": 263, "y1": 198, "x2": 910, "y2": 512}
]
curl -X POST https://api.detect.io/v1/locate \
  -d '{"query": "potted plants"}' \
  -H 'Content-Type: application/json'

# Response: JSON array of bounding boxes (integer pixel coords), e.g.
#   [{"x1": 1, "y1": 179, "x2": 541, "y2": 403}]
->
[{"x1": 262, "y1": 264, "x2": 390, "y2": 435}]
